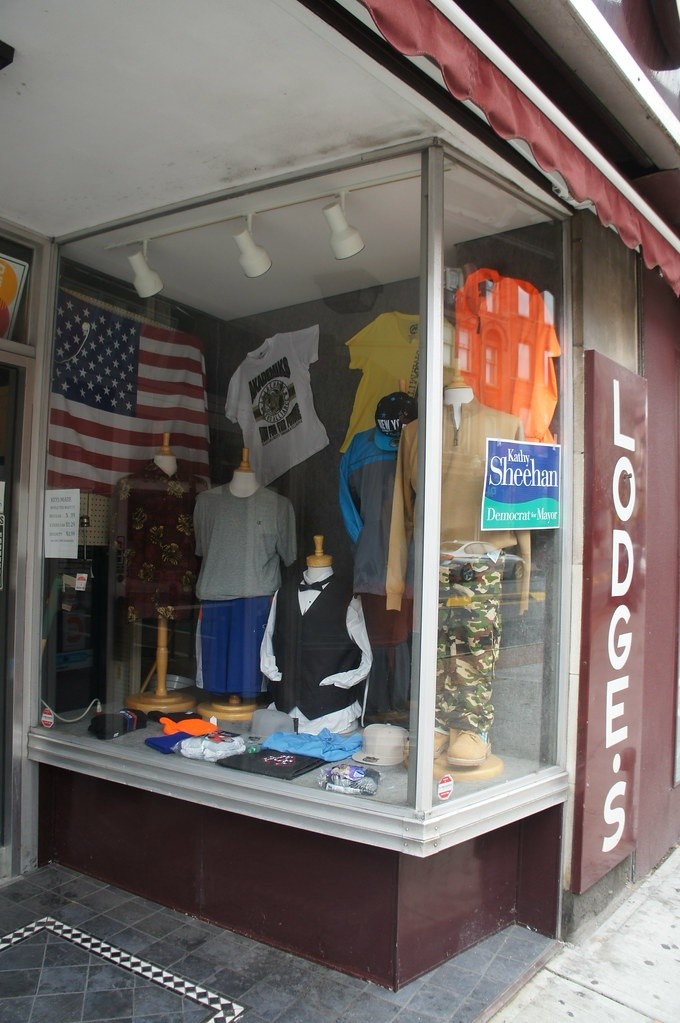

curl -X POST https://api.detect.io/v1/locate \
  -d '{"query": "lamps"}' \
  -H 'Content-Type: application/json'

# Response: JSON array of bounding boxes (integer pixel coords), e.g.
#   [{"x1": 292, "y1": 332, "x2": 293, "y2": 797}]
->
[
  {"x1": 322, "y1": 187, "x2": 366, "y2": 261},
  {"x1": 127, "y1": 238, "x2": 164, "y2": 300},
  {"x1": 78, "y1": 526, "x2": 96, "y2": 580},
  {"x1": 233, "y1": 211, "x2": 272, "y2": 278}
]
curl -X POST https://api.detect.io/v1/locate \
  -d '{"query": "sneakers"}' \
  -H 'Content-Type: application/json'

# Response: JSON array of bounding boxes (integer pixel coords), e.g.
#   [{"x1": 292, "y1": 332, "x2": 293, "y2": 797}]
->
[
  {"x1": 447, "y1": 728, "x2": 492, "y2": 766},
  {"x1": 404, "y1": 731, "x2": 450, "y2": 767}
]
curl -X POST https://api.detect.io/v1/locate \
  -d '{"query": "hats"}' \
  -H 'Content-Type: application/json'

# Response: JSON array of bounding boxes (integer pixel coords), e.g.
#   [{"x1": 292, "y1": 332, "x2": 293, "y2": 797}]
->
[
  {"x1": 235, "y1": 710, "x2": 293, "y2": 749},
  {"x1": 352, "y1": 723, "x2": 408, "y2": 766}
]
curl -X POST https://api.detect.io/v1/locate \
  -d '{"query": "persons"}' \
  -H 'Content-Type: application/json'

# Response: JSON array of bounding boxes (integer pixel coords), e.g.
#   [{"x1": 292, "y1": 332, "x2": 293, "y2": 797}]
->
[
  {"x1": 340, "y1": 387, "x2": 524, "y2": 766},
  {"x1": 116, "y1": 454, "x2": 373, "y2": 736}
]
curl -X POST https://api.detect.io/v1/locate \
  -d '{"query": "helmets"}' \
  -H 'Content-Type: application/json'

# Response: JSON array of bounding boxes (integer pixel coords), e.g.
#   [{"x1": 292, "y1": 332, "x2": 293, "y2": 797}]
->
[{"x1": 375, "y1": 392, "x2": 418, "y2": 438}]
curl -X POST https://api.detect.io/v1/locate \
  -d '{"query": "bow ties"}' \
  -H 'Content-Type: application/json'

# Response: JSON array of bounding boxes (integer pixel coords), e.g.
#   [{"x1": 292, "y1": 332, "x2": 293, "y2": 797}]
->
[{"x1": 299, "y1": 574, "x2": 335, "y2": 592}]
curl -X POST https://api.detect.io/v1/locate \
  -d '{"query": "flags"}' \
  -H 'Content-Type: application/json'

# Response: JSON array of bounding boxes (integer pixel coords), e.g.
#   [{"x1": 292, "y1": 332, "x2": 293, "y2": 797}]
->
[{"x1": 45, "y1": 288, "x2": 212, "y2": 494}]
